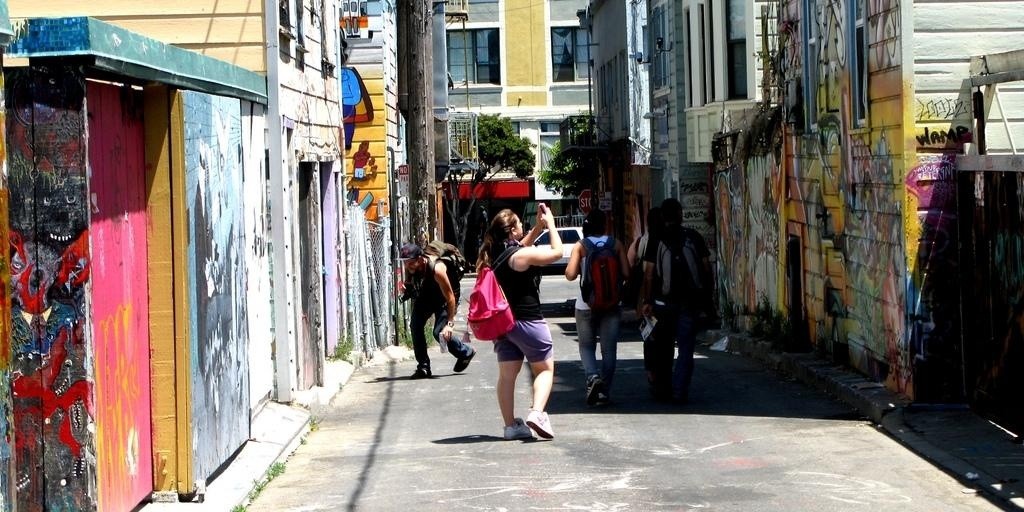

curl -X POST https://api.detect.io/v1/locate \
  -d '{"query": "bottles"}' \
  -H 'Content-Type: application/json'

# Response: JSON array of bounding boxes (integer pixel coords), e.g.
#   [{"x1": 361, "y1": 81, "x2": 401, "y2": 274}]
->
[
  {"x1": 377, "y1": 199, "x2": 385, "y2": 217},
  {"x1": 439, "y1": 332, "x2": 449, "y2": 354}
]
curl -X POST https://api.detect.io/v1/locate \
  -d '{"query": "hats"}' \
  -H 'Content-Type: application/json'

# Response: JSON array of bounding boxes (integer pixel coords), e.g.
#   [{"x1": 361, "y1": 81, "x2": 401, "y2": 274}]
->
[{"x1": 397, "y1": 243, "x2": 424, "y2": 264}]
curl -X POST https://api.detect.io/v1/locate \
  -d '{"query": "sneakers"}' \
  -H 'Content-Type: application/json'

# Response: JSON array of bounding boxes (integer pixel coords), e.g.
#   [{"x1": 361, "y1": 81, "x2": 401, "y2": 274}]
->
[
  {"x1": 504, "y1": 418, "x2": 529, "y2": 439},
  {"x1": 410, "y1": 368, "x2": 431, "y2": 379},
  {"x1": 453, "y1": 348, "x2": 477, "y2": 371},
  {"x1": 584, "y1": 373, "x2": 610, "y2": 406},
  {"x1": 525, "y1": 409, "x2": 557, "y2": 441}
]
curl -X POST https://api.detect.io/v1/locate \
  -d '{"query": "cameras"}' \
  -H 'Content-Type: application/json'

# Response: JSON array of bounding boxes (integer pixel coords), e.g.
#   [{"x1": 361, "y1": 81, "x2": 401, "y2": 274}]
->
[{"x1": 398, "y1": 285, "x2": 417, "y2": 303}]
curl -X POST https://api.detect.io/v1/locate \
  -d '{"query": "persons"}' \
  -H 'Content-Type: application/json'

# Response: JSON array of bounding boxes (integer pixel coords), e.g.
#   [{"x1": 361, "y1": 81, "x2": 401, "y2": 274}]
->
[
  {"x1": 626, "y1": 205, "x2": 662, "y2": 321},
  {"x1": 473, "y1": 204, "x2": 489, "y2": 240},
  {"x1": 564, "y1": 208, "x2": 631, "y2": 408},
  {"x1": 468, "y1": 201, "x2": 564, "y2": 443},
  {"x1": 394, "y1": 242, "x2": 477, "y2": 380},
  {"x1": 637, "y1": 197, "x2": 716, "y2": 407}
]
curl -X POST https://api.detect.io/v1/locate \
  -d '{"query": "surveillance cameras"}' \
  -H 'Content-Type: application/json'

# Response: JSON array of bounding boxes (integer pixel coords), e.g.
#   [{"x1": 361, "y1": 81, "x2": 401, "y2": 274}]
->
[
  {"x1": 655, "y1": 38, "x2": 663, "y2": 50},
  {"x1": 637, "y1": 52, "x2": 642, "y2": 62}
]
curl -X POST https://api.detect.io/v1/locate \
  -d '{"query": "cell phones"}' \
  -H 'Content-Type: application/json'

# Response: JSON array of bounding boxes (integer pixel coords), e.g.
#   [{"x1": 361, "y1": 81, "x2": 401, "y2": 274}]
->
[{"x1": 539, "y1": 205, "x2": 546, "y2": 214}]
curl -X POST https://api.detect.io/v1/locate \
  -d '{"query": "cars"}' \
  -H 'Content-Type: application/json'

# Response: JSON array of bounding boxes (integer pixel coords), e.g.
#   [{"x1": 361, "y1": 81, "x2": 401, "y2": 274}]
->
[{"x1": 520, "y1": 223, "x2": 591, "y2": 277}]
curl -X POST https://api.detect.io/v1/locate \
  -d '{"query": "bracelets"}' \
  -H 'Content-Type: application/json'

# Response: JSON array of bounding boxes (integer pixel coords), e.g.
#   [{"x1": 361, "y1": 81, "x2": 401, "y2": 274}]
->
[
  {"x1": 641, "y1": 297, "x2": 652, "y2": 305},
  {"x1": 444, "y1": 320, "x2": 454, "y2": 328}
]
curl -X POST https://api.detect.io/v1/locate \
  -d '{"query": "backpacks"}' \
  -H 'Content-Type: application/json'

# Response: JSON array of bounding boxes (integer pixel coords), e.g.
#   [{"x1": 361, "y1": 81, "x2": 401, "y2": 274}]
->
[
  {"x1": 424, "y1": 238, "x2": 466, "y2": 281},
  {"x1": 467, "y1": 245, "x2": 527, "y2": 341},
  {"x1": 579, "y1": 235, "x2": 623, "y2": 309},
  {"x1": 654, "y1": 229, "x2": 702, "y2": 304}
]
